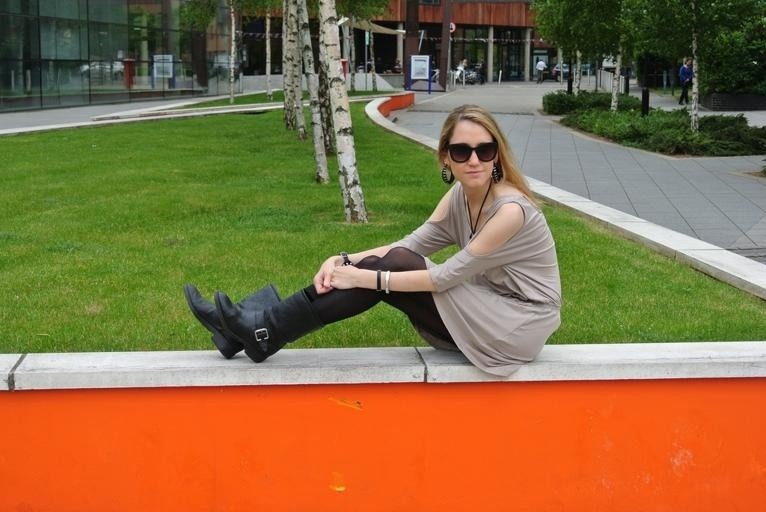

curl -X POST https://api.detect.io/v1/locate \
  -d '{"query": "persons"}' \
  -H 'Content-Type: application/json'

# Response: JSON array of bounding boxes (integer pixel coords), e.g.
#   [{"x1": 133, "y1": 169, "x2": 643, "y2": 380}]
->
[
  {"x1": 678, "y1": 56, "x2": 693, "y2": 105},
  {"x1": 536, "y1": 58, "x2": 548, "y2": 84},
  {"x1": 463, "y1": 59, "x2": 468, "y2": 69},
  {"x1": 391, "y1": 59, "x2": 402, "y2": 74},
  {"x1": 184, "y1": 103, "x2": 560, "y2": 376},
  {"x1": 478, "y1": 59, "x2": 485, "y2": 85},
  {"x1": 375, "y1": 57, "x2": 385, "y2": 74}
]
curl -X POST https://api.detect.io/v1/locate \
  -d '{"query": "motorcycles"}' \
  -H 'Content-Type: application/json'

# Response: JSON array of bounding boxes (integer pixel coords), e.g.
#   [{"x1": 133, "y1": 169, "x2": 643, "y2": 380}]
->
[{"x1": 455, "y1": 59, "x2": 487, "y2": 84}]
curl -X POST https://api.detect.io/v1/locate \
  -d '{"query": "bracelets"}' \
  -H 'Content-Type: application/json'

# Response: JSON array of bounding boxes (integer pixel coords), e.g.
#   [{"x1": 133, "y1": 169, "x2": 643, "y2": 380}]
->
[
  {"x1": 385, "y1": 271, "x2": 390, "y2": 294},
  {"x1": 377, "y1": 269, "x2": 381, "y2": 292},
  {"x1": 340, "y1": 251, "x2": 351, "y2": 265}
]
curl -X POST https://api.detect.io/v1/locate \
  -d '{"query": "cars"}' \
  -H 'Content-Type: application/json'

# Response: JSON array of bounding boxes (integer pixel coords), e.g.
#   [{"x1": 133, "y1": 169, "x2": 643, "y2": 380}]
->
[
  {"x1": 180, "y1": 51, "x2": 242, "y2": 83},
  {"x1": 79, "y1": 61, "x2": 124, "y2": 80},
  {"x1": 543, "y1": 63, "x2": 595, "y2": 82}
]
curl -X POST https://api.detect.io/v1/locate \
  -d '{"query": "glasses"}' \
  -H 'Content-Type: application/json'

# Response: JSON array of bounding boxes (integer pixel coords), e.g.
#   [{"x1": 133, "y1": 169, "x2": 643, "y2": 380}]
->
[{"x1": 446, "y1": 142, "x2": 497, "y2": 163}]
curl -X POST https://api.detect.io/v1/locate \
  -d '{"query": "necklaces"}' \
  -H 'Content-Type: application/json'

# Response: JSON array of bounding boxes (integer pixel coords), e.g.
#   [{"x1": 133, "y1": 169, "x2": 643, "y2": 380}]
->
[{"x1": 467, "y1": 179, "x2": 492, "y2": 234}]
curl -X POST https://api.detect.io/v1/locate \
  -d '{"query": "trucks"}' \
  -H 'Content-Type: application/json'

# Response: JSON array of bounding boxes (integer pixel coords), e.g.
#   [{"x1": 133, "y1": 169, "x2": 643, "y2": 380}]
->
[{"x1": 601, "y1": 52, "x2": 636, "y2": 78}]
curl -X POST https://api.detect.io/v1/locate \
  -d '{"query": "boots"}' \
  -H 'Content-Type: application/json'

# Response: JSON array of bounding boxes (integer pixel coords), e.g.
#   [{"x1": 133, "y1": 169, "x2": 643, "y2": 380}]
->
[{"x1": 183, "y1": 284, "x2": 324, "y2": 362}]
